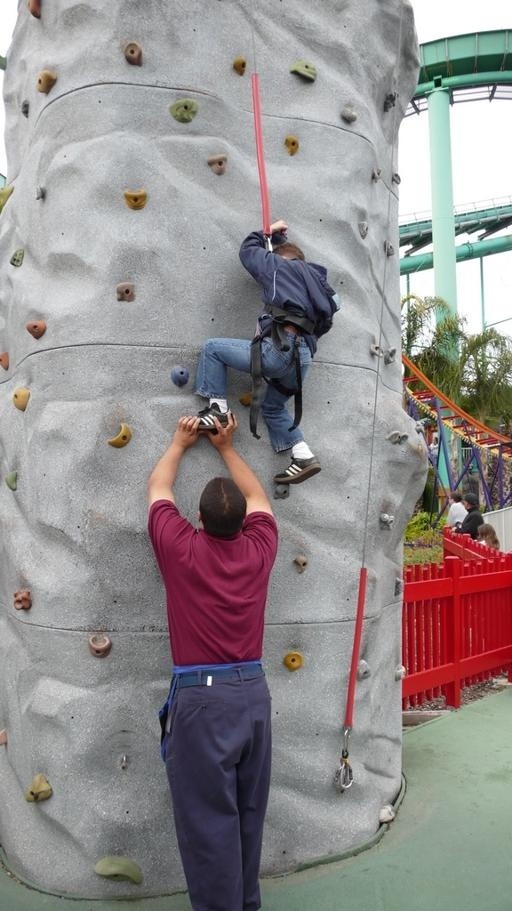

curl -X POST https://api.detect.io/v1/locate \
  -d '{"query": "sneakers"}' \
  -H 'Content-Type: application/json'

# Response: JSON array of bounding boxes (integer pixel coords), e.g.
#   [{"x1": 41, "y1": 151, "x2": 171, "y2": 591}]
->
[
  {"x1": 274, "y1": 457, "x2": 321, "y2": 484},
  {"x1": 198, "y1": 403, "x2": 233, "y2": 429}
]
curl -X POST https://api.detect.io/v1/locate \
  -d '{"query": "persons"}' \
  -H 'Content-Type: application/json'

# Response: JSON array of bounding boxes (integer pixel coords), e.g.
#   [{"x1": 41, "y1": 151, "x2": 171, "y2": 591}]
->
[
  {"x1": 447, "y1": 493, "x2": 469, "y2": 529},
  {"x1": 474, "y1": 524, "x2": 500, "y2": 557},
  {"x1": 192, "y1": 220, "x2": 340, "y2": 484},
  {"x1": 452, "y1": 493, "x2": 484, "y2": 543},
  {"x1": 147, "y1": 411, "x2": 280, "y2": 911}
]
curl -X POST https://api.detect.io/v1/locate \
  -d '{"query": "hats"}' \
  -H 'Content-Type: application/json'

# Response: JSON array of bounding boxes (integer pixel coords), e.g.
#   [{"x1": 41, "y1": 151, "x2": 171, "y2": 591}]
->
[{"x1": 460, "y1": 493, "x2": 477, "y2": 504}]
